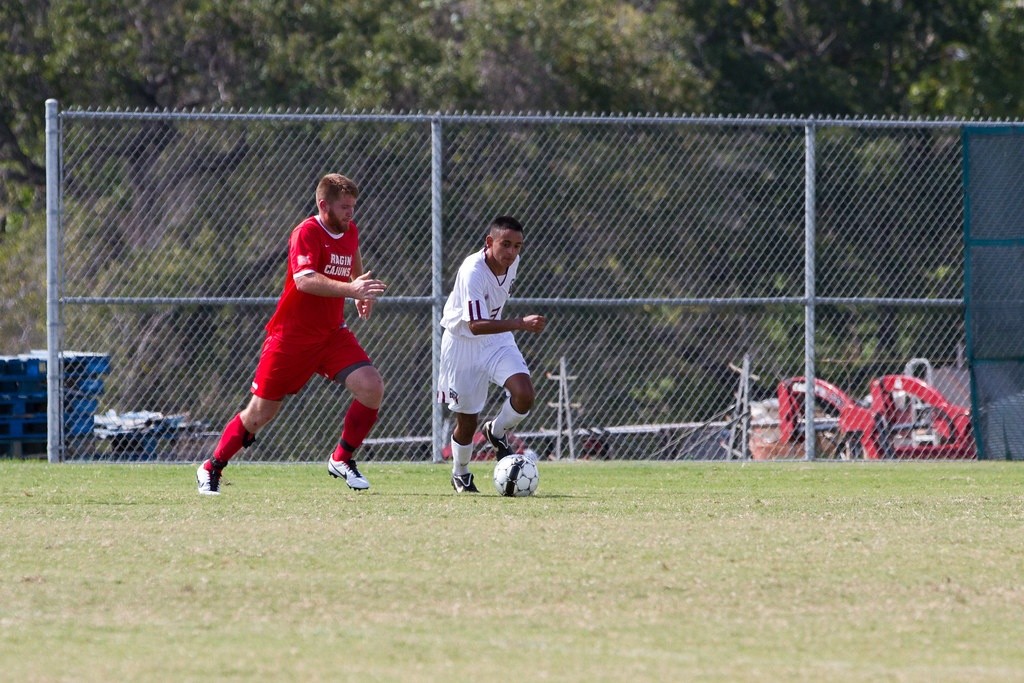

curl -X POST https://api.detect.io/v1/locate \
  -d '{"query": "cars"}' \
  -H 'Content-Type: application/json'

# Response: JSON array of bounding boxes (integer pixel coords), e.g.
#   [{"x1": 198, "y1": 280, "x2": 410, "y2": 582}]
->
[{"x1": 737, "y1": 400, "x2": 861, "y2": 460}]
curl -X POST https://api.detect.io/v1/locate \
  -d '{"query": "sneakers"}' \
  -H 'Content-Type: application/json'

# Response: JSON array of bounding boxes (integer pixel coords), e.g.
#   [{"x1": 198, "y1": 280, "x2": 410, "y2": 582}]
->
[
  {"x1": 197, "y1": 459, "x2": 222, "y2": 496},
  {"x1": 482, "y1": 421, "x2": 515, "y2": 462},
  {"x1": 451, "y1": 473, "x2": 479, "y2": 492},
  {"x1": 327, "y1": 453, "x2": 371, "y2": 491}
]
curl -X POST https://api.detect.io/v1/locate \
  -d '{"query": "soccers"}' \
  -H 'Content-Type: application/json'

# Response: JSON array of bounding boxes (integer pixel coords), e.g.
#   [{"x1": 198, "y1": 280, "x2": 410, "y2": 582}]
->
[{"x1": 493, "y1": 454, "x2": 540, "y2": 497}]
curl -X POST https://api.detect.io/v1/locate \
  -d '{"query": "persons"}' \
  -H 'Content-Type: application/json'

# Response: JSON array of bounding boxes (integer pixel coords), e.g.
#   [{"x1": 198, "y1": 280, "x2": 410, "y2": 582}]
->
[
  {"x1": 435, "y1": 215, "x2": 547, "y2": 494},
  {"x1": 194, "y1": 172, "x2": 388, "y2": 499}
]
